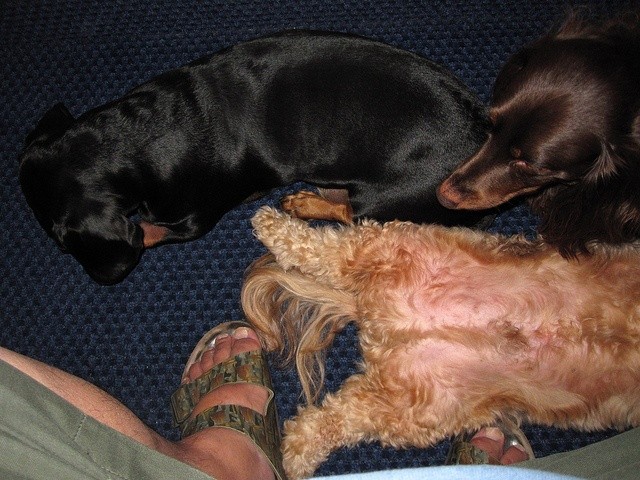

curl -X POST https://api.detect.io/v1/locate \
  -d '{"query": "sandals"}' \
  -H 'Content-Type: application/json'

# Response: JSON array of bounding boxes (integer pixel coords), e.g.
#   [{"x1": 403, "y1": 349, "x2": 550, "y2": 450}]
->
[
  {"x1": 452, "y1": 416, "x2": 535, "y2": 466},
  {"x1": 170, "y1": 321, "x2": 287, "y2": 480}
]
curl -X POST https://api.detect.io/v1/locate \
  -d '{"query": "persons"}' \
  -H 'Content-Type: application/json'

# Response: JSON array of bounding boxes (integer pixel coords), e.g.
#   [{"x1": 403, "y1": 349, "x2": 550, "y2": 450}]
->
[{"x1": 0, "y1": 321, "x2": 640, "y2": 480}]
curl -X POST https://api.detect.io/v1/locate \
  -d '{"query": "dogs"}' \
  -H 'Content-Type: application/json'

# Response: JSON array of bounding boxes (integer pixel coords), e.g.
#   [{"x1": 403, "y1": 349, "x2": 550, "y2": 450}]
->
[
  {"x1": 240, "y1": 203, "x2": 640, "y2": 480},
  {"x1": 20, "y1": 27, "x2": 497, "y2": 287},
  {"x1": 434, "y1": 0, "x2": 640, "y2": 264}
]
